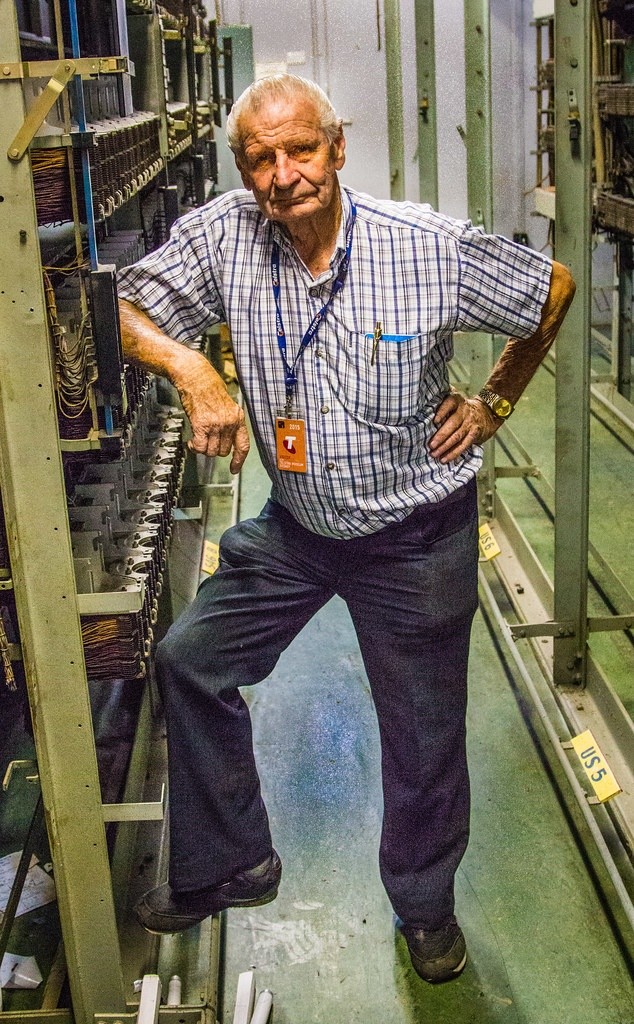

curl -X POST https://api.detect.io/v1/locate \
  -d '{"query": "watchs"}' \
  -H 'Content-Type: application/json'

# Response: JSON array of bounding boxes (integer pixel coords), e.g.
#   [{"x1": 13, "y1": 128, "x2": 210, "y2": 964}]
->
[{"x1": 478, "y1": 388, "x2": 515, "y2": 420}]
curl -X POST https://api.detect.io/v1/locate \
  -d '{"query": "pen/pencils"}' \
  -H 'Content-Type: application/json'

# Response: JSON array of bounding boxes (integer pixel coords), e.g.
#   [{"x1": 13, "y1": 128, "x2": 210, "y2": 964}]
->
[{"x1": 368, "y1": 320, "x2": 383, "y2": 366}]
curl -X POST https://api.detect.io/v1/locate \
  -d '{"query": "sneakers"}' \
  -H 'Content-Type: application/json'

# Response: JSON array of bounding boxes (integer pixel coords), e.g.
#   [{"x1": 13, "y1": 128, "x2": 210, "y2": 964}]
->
[
  {"x1": 135, "y1": 846, "x2": 280, "y2": 935},
  {"x1": 398, "y1": 910, "x2": 468, "y2": 980}
]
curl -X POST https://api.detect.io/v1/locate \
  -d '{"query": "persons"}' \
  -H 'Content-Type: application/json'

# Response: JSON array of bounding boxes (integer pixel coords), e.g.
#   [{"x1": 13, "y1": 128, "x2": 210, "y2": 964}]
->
[{"x1": 113, "y1": 73, "x2": 579, "y2": 982}]
